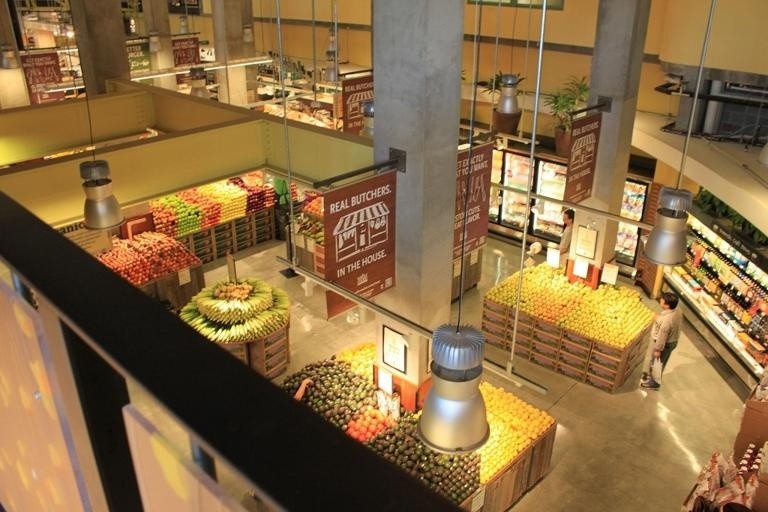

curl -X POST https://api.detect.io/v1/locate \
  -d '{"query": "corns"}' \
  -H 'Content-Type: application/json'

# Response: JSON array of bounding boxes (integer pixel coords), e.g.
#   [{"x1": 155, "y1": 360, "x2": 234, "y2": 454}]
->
[{"x1": 179, "y1": 279, "x2": 289, "y2": 343}]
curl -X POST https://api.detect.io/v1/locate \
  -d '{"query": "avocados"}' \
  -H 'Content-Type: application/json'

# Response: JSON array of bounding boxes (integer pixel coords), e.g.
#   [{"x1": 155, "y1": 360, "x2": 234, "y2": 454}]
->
[
  {"x1": 365, "y1": 408, "x2": 480, "y2": 504},
  {"x1": 282, "y1": 355, "x2": 377, "y2": 431}
]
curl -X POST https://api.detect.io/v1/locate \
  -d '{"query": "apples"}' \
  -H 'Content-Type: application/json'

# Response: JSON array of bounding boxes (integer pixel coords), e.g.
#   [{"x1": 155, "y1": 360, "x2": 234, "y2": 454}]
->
[
  {"x1": 177, "y1": 188, "x2": 221, "y2": 229},
  {"x1": 162, "y1": 193, "x2": 201, "y2": 238},
  {"x1": 151, "y1": 204, "x2": 176, "y2": 239},
  {"x1": 96, "y1": 230, "x2": 201, "y2": 286}
]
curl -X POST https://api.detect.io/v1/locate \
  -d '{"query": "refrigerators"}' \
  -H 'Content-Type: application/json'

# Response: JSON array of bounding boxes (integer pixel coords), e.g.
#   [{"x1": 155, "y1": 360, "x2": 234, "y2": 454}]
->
[
  {"x1": 501, "y1": 135, "x2": 532, "y2": 245},
  {"x1": 614, "y1": 164, "x2": 652, "y2": 277},
  {"x1": 532, "y1": 149, "x2": 570, "y2": 251},
  {"x1": 474, "y1": 130, "x2": 500, "y2": 241}
]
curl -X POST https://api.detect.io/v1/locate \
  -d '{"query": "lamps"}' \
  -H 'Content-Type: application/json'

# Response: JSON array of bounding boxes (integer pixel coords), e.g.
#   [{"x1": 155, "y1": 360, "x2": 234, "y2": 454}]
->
[
  {"x1": 413, "y1": 0, "x2": 490, "y2": 456},
  {"x1": 323, "y1": 0, "x2": 341, "y2": 81},
  {"x1": 495, "y1": 0, "x2": 520, "y2": 115},
  {"x1": 643, "y1": 0, "x2": 720, "y2": 266},
  {"x1": 188, "y1": 0, "x2": 210, "y2": 91},
  {"x1": 68, "y1": 0, "x2": 126, "y2": 231}
]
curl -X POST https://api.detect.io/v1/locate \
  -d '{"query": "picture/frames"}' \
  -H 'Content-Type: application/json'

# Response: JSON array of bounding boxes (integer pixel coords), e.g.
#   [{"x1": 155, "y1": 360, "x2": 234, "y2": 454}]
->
[
  {"x1": 382, "y1": 323, "x2": 406, "y2": 375},
  {"x1": 425, "y1": 336, "x2": 432, "y2": 373}
]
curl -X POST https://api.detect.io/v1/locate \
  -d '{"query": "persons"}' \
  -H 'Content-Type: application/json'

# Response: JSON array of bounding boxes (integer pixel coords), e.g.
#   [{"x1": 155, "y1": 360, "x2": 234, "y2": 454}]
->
[
  {"x1": 640, "y1": 290, "x2": 683, "y2": 388},
  {"x1": 552, "y1": 208, "x2": 575, "y2": 273}
]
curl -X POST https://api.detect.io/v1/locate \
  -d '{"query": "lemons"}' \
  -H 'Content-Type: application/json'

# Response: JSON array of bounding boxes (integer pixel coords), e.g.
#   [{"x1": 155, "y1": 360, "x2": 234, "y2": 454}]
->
[
  {"x1": 481, "y1": 381, "x2": 555, "y2": 482},
  {"x1": 199, "y1": 180, "x2": 248, "y2": 224},
  {"x1": 486, "y1": 262, "x2": 655, "y2": 348},
  {"x1": 336, "y1": 344, "x2": 377, "y2": 383}
]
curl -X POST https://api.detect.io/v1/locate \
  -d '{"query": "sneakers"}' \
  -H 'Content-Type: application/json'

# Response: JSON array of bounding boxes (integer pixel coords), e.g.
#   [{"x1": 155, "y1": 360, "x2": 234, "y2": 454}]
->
[
  {"x1": 642, "y1": 375, "x2": 651, "y2": 380},
  {"x1": 641, "y1": 379, "x2": 660, "y2": 388}
]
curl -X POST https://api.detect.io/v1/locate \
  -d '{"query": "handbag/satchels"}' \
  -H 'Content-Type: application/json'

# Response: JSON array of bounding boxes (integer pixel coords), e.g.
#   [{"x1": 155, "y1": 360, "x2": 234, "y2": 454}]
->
[{"x1": 651, "y1": 356, "x2": 663, "y2": 385}]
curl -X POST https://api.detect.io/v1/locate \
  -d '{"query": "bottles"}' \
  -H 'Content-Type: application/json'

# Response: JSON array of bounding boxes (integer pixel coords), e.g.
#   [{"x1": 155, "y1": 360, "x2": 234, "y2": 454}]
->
[{"x1": 680, "y1": 231, "x2": 768, "y2": 348}]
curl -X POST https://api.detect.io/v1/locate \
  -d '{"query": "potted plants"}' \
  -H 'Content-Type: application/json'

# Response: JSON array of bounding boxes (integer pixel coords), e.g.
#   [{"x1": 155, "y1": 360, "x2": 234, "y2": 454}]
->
[
  {"x1": 544, "y1": 74, "x2": 590, "y2": 158},
  {"x1": 480, "y1": 70, "x2": 525, "y2": 135}
]
